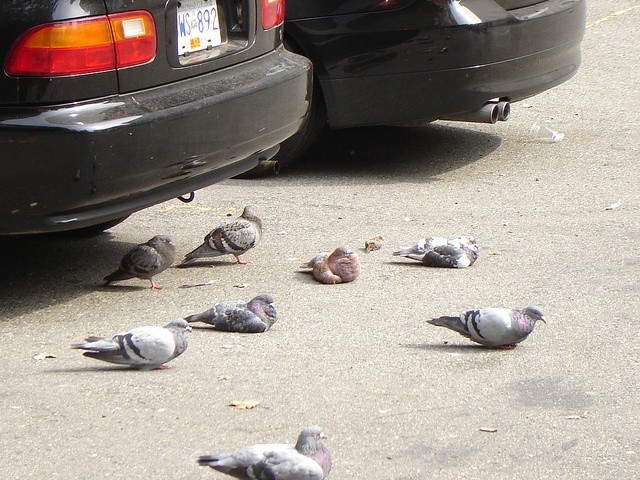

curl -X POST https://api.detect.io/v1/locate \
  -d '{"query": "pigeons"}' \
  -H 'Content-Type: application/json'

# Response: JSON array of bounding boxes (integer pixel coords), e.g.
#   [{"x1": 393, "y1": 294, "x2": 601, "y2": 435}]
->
[
  {"x1": 195, "y1": 425, "x2": 332, "y2": 479},
  {"x1": 426, "y1": 306, "x2": 547, "y2": 349},
  {"x1": 183, "y1": 295, "x2": 277, "y2": 333},
  {"x1": 181, "y1": 205, "x2": 262, "y2": 266},
  {"x1": 299, "y1": 246, "x2": 360, "y2": 284},
  {"x1": 102, "y1": 235, "x2": 176, "y2": 290},
  {"x1": 393, "y1": 234, "x2": 479, "y2": 269},
  {"x1": 69, "y1": 318, "x2": 193, "y2": 371}
]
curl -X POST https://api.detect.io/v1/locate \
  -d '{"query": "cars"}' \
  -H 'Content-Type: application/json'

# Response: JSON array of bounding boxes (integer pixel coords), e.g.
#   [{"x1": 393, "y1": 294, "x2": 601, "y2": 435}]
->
[
  {"x1": 230, "y1": 1, "x2": 586, "y2": 179},
  {"x1": 0, "y1": 0, "x2": 312, "y2": 236}
]
